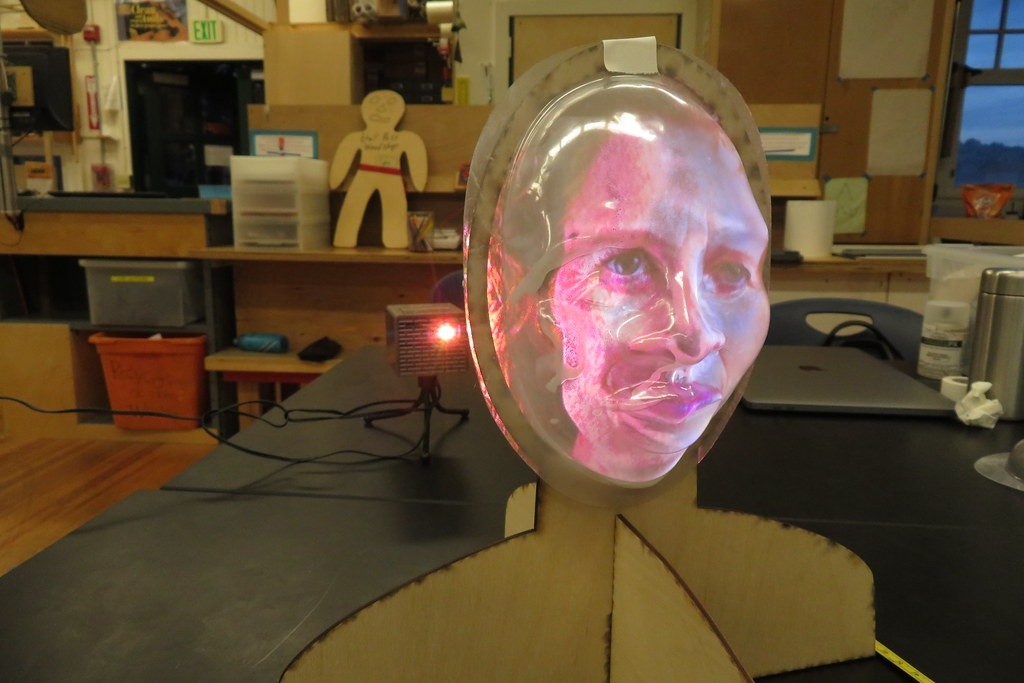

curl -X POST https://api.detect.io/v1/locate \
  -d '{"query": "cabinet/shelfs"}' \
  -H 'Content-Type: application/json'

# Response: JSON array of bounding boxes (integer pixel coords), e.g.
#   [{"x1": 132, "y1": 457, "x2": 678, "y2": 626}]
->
[{"x1": 183, "y1": 245, "x2": 462, "y2": 435}]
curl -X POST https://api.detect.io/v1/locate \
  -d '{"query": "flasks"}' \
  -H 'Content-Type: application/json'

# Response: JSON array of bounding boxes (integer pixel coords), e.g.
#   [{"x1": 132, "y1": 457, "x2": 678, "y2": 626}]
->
[{"x1": 970, "y1": 268, "x2": 1024, "y2": 419}]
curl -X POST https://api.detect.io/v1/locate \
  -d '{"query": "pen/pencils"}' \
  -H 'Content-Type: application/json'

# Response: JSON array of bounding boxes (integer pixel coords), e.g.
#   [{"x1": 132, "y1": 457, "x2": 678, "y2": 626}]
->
[{"x1": 408, "y1": 212, "x2": 434, "y2": 252}]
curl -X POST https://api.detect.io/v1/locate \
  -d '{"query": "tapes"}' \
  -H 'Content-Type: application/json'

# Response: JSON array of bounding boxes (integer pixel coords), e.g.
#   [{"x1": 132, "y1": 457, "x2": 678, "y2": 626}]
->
[{"x1": 940, "y1": 375, "x2": 968, "y2": 403}]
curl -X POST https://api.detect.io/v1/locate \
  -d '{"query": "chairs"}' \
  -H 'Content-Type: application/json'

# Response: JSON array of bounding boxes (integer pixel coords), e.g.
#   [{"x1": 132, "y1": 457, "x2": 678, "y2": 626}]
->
[{"x1": 765, "y1": 297, "x2": 924, "y2": 360}]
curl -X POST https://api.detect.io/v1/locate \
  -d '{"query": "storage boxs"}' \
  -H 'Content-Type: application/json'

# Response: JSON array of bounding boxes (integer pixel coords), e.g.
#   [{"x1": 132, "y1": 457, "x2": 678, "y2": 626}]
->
[
  {"x1": 232, "y1": 156, "x2": 331, "y2": 250},
  {"x1": 76, "y1": 257, "x2": 204, "y2": 327},
  {"x1": 89, "y1": 333, "x2": 206, "y2": 432}
]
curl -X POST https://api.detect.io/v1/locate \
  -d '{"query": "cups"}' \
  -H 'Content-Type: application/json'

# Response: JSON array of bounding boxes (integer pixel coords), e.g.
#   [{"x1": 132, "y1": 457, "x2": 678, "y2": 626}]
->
[
  {"x1": 407, "y1": 211, "x2": 434, "y2": 252},
  {"x1": 918, "y1": 301, "x2": 970, "y2": 379},
  {"x1": 785, "y1": 199, "x2": 835, "y2": 259}
]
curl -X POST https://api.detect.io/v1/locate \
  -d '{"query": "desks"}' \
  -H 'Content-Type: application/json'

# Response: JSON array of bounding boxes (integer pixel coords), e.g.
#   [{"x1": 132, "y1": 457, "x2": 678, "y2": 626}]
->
[{"x1": 0, "y1": 343, "x2": 1024, "y2": 683}]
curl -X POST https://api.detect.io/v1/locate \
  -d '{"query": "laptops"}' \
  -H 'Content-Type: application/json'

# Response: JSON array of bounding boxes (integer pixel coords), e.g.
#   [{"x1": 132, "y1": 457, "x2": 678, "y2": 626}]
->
[{"x1": 740, "y1": 346, "x2": 958, "y2": 418}]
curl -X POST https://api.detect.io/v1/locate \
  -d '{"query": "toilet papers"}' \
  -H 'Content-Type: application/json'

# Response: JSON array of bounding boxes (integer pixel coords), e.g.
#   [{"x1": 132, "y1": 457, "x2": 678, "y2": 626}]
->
[
  {"x1": 784, "y1": 200, "x2": 834, "y2": 257},
  {"x1": 940, "y1": 376, "x2": 968, "y2": 402}
]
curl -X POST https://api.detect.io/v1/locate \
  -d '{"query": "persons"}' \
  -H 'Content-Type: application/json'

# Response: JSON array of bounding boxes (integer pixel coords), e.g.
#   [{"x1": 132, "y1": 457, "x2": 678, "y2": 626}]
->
[{"x1": 547, "y1": 110, "x2": 772, "y2": 486}]
